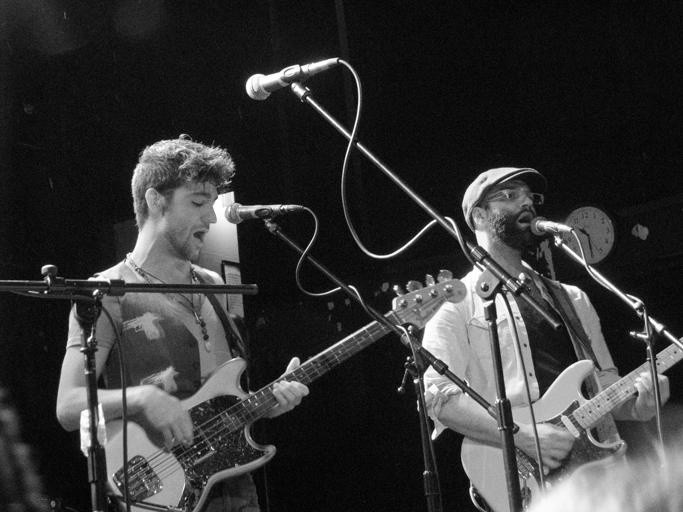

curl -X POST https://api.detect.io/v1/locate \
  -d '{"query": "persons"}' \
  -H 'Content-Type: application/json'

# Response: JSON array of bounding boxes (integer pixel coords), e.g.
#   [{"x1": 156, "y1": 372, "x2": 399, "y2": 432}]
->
[
  {"x1": 54, "y1": 137, "x2": 310, "y2": 511},
  {"x1": 421, "y1": 162, "x2": 671, "y2": 512}
]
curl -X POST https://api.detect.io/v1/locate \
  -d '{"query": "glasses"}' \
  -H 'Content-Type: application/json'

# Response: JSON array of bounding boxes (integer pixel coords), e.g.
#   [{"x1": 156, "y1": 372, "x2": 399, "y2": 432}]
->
[{"x1": 484, "y1": 189, "x2": 544, "y2": 206}]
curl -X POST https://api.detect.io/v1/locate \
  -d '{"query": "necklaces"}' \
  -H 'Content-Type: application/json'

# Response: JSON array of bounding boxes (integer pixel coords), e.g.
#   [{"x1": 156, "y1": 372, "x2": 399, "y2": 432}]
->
[{"x1": 126, "y1": 252, "x2": 211, "y2": 353}]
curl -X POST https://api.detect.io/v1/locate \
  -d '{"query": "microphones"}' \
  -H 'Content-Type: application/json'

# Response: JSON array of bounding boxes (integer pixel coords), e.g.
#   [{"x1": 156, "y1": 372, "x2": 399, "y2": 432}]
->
[
  {"x1": 224, "y1": 201, "x2": 304, "y2": 224},
  {"x1": 529, "y1": 215, "x2": 574, "y2": 237},
  {"x1": 245, "y1": 56, "x2": 340, "y2": 102}
]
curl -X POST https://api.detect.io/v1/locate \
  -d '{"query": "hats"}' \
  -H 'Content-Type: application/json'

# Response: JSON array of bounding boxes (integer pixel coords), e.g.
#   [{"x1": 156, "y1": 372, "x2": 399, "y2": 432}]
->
[{"x1": 462, "y1": 167, "x2": 547, "y2": 233}]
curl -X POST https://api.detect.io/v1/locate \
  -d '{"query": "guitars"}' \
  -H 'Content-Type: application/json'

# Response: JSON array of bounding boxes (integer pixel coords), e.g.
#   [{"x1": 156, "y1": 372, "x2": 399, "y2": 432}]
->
[
  {"x1": 460, "y1": 333, "x2": 683, "y2": 512},
  {"x1": 101, "y1": 268, "x2": 468, "y2": 512}
]
